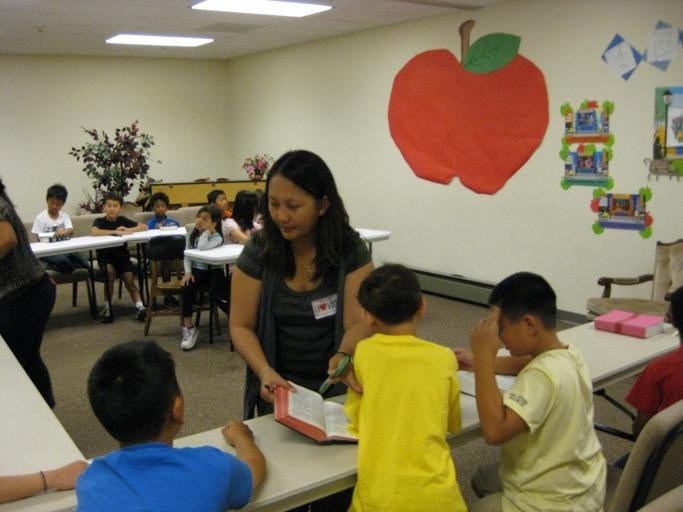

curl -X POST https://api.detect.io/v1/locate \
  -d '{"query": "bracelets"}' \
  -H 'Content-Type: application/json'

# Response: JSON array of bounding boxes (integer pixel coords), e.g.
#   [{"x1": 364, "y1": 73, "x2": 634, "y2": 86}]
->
[
  {"x1": 39, "y1": 471, "x2": 48, "y2": 491},
  {"x1": 336, "y1": 351, "x2": 352, "y2": 359}
]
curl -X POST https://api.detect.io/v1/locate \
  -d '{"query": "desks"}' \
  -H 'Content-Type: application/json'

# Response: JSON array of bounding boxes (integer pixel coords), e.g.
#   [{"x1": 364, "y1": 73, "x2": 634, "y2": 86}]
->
[
  {"x1": 2, "y1": 338, "x2": 88, "y2": 511},
  {"x1": 184, "y1": 228, "x2": 392, "y2": 352},
  {"x1": 173, "y1": 321, "x2": 681, "y2": 512}
]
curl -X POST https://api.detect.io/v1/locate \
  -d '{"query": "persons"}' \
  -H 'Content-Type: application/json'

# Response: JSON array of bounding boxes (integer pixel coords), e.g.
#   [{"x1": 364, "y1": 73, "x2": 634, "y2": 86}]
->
[
  {"x1": 91, "y1": 194, "x2": 150, "y2": 321},
  {"x1": 147, "y1": 192, "x2": 181, "y2": 307},
  {"x1": 0, "y1": 460, "x2": 91, "y2": 504},
  {"x1": 0, "y1": 180, "x2": 57, "y2": 409},
  {"x1": 228, "y1": 150, "x2": 377, "y2": 421},
  {"x1": 452, "y1": 273, "x2": 607, "y2": 512},
  {"x1": 626, "y1": 286, "x2": 683, "y2": 432},
  {"x1": 344, "y1": 262, "x2": 469, "y2": 512},
  {"x1": 181, "y1": 187, "x2": 264, "y2": 351},
  {"x1": 30, "y1": 184, "x2": 89, "y2": 278},
  {"x1": 76, "y1": 340, "x2": 267, "y2": 512}
]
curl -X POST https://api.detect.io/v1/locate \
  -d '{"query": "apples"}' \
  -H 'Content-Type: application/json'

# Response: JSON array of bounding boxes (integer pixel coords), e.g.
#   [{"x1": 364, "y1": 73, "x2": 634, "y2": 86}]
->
[{"x1": 388, "y1": 19, "x2": 549, "y2": 195}]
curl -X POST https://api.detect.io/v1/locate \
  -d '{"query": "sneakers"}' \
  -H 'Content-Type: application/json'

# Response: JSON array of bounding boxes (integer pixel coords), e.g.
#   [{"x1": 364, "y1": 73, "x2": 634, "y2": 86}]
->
[
  {"x1": 99, "y1": 306, "x2": 111, "y2": 321},
  {"x1": 163, "y1": 295, "x2": 180, "y2": 307},
  {"x1": 135, "y1": 305, "x2": 148, "y2": 322},
  {"x1": 181, "y1": 324, "x2": 201, "y2": 350}
]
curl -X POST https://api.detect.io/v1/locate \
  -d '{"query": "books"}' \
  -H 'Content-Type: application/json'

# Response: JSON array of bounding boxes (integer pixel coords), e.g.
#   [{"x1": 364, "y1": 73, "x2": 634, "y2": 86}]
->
[{"x1": 273, "y1": 380, "x2": 358, "y2": 445}]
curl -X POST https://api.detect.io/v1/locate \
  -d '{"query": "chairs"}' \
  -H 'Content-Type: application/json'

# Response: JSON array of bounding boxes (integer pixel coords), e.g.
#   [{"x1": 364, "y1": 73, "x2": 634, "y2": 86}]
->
[
  {"x1": 586, "y1": 239, "x2": 683, "y2": 334},
  {"x1": 23, "y1": 206, "x2": 223, "y2": 336}
]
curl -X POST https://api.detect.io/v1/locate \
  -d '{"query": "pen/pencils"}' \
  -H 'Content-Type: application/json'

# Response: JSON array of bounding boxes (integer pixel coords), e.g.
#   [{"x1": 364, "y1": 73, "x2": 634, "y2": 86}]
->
[{"x1": 319, "y1": 357, "x2": 350, "y2": 394}]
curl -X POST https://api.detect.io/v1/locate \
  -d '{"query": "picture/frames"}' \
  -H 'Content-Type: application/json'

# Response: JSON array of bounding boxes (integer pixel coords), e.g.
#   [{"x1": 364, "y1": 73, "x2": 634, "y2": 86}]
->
[
  {"x1": 565, "y1": 109, "x2": 608, "y2": 143},
  {"x1": 653, "y1": 86, "x2": 683, "y2": 160},
  {"x1": 599, "y1": 194, "x2": 645, "y2": 230},
  {"x1": 564, "y1": 151, "x2": 608, "y2": 186}
]
